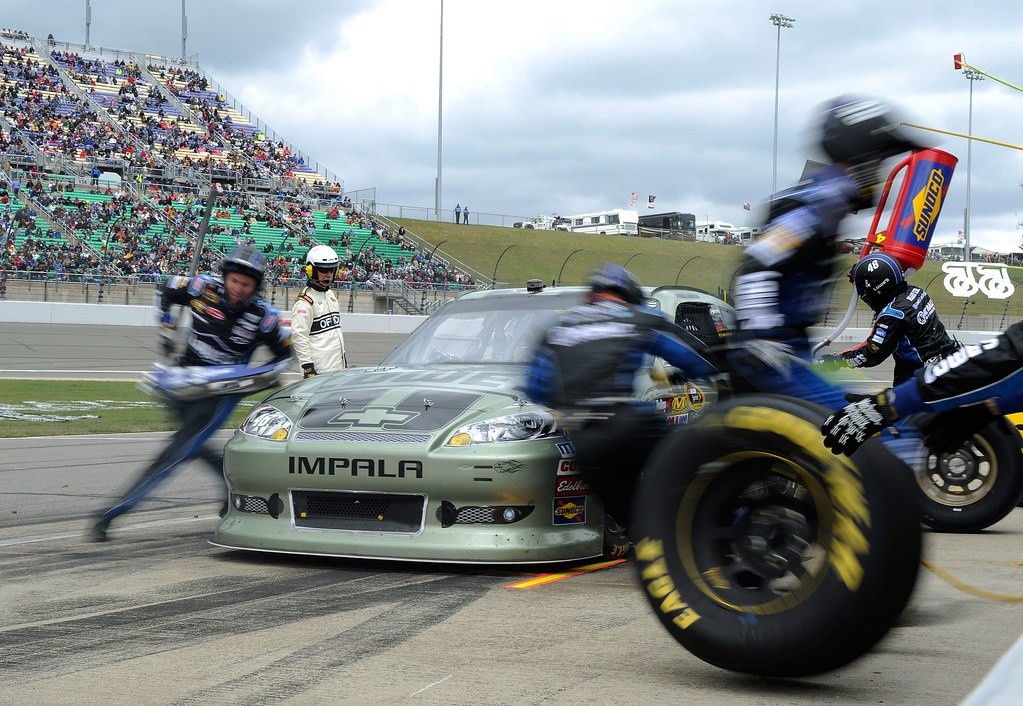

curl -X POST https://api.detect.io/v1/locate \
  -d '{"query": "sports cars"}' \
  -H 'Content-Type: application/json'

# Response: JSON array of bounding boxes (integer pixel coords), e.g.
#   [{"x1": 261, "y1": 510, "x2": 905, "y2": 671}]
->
[{"x1": 210, "y1": 287, "x2": 742, "y2": 574}]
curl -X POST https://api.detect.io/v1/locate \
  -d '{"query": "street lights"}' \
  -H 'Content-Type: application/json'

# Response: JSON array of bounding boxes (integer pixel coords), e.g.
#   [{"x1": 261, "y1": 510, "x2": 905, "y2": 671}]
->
[
  {"x1": 767, "y1": 10, "x2": 796, "y2": 228},
  {"x1": 960, "y1": 67, "x2": 985, "y2": 267}
]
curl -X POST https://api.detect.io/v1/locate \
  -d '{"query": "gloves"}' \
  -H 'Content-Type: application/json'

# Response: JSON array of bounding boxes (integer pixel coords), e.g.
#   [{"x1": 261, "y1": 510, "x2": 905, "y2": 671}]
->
[
  {"x1": 820, "y1": 354, "x2": 841, "y2": 372},
  {"x1": 821, "y1": 387, "x2": 898, "y2": 458}
]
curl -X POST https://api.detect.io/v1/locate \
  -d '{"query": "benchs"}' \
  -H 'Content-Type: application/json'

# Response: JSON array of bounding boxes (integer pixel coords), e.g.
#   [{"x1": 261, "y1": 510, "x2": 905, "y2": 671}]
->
[{"x1": 0, "y1": 48, "x2": 463, "y2": 289}]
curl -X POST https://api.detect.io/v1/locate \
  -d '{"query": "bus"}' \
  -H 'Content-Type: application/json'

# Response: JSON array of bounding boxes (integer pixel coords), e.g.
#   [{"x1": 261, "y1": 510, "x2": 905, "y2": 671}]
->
[
  {"x1": 697, "y1": 223, "x2": 738, "y2": 244},
  {"x1": 527, "y1": 210, "x2": 639, "y2": 238},
  {"x1": 634, "y1": 211, "x2": 696, "y2": 242}
]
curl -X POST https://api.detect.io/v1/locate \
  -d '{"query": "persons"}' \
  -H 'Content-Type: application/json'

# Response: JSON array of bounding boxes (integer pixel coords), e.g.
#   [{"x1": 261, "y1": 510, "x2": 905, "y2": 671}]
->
[
  {"x1": 724, "y1": 94, "x2": 929, "y2": 429},
  {"x1": 82, "y1": 244, "x2": 294, "y2": 544},
  {"x1": 820, "y1": 319, "x2": 1023, "y2": 457},
  {"x1": 0, "y1": 27, "x2": 475, "y2": 302},
  {"x1": 814, "y1": 251, "x2": 957, "y2": 390},
  {"x1": 463, "y1": 207, "x2": 469, "y2": 225},
  {"x1": 524, "y1": 265, "x2": 720, "y2": 530},
  {"x1": 454, "y1": 203, "x2": 461, "y2": 224},
  {"x1": 291, "y1": 245, "x2": 348, "y2": 380}
]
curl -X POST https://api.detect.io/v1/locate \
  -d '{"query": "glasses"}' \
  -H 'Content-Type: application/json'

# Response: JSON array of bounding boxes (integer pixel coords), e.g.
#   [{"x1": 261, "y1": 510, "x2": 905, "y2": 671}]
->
[{"x1": 316, "y1": 266, "x2": 337, "y2": 274}]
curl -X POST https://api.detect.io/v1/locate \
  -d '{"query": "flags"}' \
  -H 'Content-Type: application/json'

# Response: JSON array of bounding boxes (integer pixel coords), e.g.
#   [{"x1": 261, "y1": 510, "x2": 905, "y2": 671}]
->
[
  {"x1": 648, "y1": 194, "x2": 656, "y2": 209},
  {"x1": 742, "y1": 202, "x2": 751, "y2": 211},
  {"x1": 630, "y1": 192, "x2": 638, "y2": 206}
]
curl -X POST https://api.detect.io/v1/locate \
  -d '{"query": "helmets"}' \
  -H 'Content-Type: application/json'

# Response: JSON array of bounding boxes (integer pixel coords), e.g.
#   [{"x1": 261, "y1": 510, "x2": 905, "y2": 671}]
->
[
  {"x1": 303, "y1": 245, "x2": 339, "y2": 279},
  {"x1": 590, "y1": 264, "x2": 645, "y2": 302},
  {"x1": 218, "y1": 245, "x2": 266, "y2": 281},
  {"x1": 846, "y1": 252, "x2": 907, "y2": 311},
  {"x1": 816, "y1": 94, "x2": 943, "y2": 166}
]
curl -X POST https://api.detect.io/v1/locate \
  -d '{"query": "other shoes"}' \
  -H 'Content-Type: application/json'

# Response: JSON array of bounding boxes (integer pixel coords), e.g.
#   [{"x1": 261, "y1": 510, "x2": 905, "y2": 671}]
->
[
  {"x1": 89, "y1": 514, "x2": 110, "y2": 542},
  {"x1": 219, "y1": 476, "x2": 230, "y2": 519}
]
